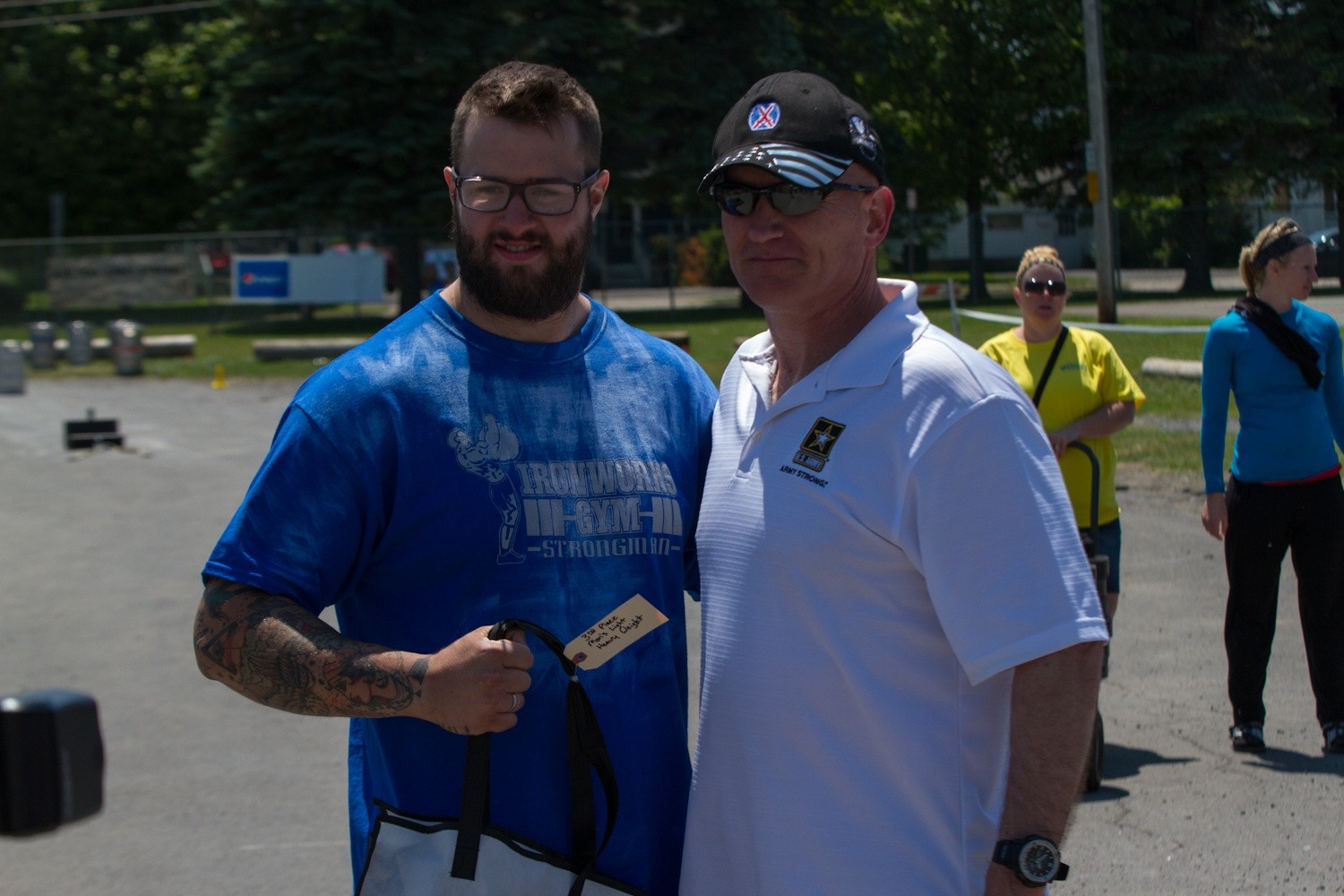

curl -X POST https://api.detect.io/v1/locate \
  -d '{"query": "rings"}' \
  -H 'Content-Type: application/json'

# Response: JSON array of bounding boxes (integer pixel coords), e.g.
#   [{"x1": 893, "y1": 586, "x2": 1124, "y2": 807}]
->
[
  {"x1": 1055, "y1": 446, "x2": 1060, "y2": 450},
  {"x1": 509, "y1": 694, "x2": 518, "y2": 713}
]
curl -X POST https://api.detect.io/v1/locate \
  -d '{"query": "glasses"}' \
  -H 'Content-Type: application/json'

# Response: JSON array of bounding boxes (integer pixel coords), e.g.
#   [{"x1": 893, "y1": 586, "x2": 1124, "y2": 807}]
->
[
  {"x1": 709, "y1": 181, "x2": 876, "y2": 217},
  {"x1": 1022, "y1": 281, "x2": 1066, "y2": 296},
  {"x1": 452, "y1": 166, "x2": 600, "y2": 216}
]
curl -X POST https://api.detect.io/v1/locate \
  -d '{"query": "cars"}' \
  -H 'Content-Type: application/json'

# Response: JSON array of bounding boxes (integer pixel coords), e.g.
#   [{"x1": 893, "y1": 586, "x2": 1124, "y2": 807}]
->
[{"x1": 1306, "y1": 228, "x2": 1342, "y2": 280}]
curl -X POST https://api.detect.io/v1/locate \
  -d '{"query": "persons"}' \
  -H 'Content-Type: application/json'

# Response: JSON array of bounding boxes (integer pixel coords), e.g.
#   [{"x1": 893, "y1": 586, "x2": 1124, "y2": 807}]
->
[
  {"x1": 976, "y1": 246, "x2": 1145, "y2": 616},
  {"x1": 192, "y1": 61, "x2": 721, "y2": 896},
  {"x1": 678, "y1": 71, "x2": 1111, "y2": 896},
  {"x1": 1201, "y1": 218, "x2": 1344, "y2": 751}
]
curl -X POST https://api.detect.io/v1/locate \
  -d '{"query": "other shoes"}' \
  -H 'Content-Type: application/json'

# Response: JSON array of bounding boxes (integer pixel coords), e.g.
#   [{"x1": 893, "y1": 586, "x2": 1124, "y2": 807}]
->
[
  {"x1": 1229, "y1": 720, "x2": 1268, "y2": 754},
  {"x1": 1321, "y1": 719, "x2": 1344, "y2": 754}
]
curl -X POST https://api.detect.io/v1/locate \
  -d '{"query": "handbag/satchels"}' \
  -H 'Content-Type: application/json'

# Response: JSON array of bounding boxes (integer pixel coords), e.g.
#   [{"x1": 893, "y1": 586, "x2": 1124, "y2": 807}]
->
[{"x1": 356, "y1": 619, "x2": 649, "y2": 896}]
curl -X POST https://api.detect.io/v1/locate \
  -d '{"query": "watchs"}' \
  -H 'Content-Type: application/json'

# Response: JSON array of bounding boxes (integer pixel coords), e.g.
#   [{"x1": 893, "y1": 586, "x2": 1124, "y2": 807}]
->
[{"x1": 991, "y1": 834, "x2": 1070, "y2": 888}]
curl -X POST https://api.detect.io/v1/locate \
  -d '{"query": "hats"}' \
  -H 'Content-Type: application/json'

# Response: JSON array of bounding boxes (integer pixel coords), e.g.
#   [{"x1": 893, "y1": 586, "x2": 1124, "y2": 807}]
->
[{"x1": 697, "y1": 71, "x2": 885, "y2": 195}]
